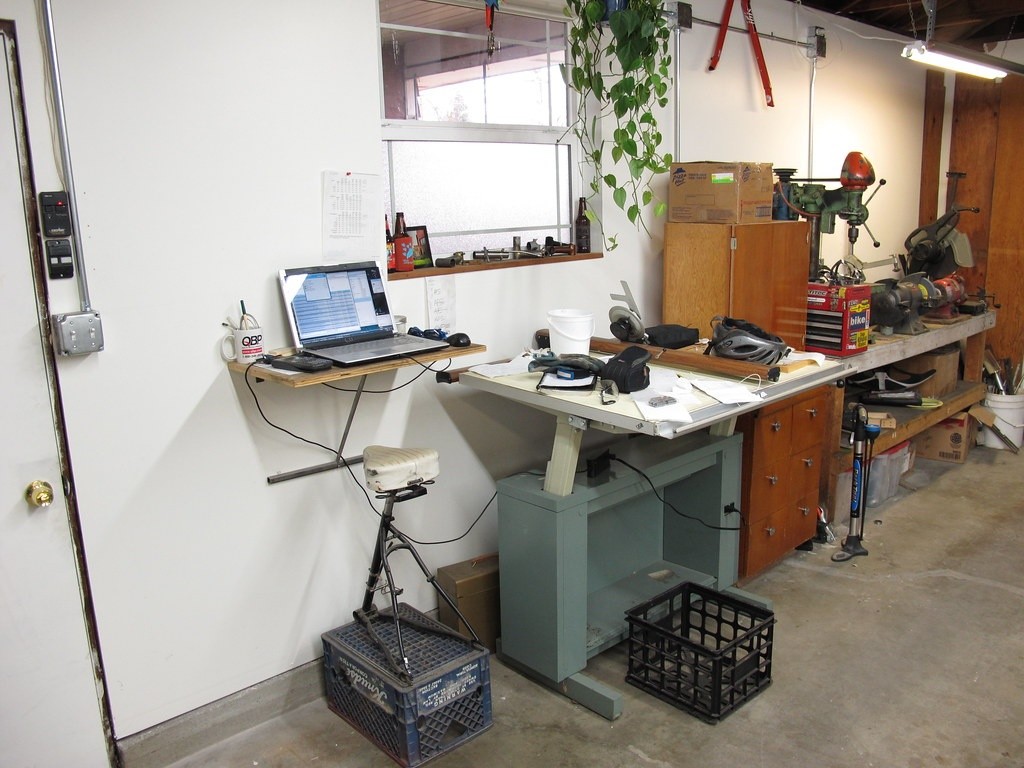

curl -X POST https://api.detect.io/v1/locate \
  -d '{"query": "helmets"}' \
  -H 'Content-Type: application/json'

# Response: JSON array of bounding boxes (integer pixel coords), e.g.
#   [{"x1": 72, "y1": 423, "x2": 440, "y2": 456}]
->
[{"x1": 703, "y1": 315, "x2": 787, "y2": 365}]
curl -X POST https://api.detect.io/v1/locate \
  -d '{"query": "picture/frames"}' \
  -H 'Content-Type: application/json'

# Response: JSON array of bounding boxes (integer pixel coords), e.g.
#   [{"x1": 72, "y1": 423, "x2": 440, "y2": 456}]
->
[{"x1": 406, "y1": 225, "x2": 434, "y2": 269}]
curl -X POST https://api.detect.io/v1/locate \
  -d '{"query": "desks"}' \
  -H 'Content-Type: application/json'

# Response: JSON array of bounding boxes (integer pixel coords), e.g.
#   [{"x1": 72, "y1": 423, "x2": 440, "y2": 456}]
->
[
  {"x1": 459, "y1": 337, "x2": 861, "y2": 722},
  {"x1": 228, "y1": 343, "x2": 486, "y2": 388}
]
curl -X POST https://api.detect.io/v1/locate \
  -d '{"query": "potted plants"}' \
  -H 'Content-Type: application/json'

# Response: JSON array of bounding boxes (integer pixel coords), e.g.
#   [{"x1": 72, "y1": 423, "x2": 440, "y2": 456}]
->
[{"x1": 556, "y1": 0, "x2": 672, "y2": 251}]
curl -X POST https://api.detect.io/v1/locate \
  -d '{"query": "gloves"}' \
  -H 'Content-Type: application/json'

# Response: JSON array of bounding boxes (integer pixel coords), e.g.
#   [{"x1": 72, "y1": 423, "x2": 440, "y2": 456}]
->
[{"x1": 528, "y1": 351, "x2": 605, "y2": 371}]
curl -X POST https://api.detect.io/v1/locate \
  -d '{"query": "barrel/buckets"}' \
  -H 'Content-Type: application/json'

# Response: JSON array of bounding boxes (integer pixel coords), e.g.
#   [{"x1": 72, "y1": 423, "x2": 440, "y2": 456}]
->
[
  {"x1": 547, "y1": 309, "x2": 596, "y2": 358},
  {"x1": 983, "y1": 387, "x2": 1024, "y2": 450}
]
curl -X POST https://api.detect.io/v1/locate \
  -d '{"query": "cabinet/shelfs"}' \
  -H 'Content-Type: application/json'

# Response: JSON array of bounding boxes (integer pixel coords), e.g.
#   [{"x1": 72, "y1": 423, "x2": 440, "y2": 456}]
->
[
  {"x1": 827, "y1": 309, "x2": 996, "y2": 524},
  {"x1": 665, "y1": 218, "x2": 834, "y2": 586}
]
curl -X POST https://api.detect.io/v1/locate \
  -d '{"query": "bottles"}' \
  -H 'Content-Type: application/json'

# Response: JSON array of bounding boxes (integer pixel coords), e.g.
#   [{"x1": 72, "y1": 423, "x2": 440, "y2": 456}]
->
[
  {"x1": 385, "y1": 214, "x2": 397, "y2": 274},
  {"x1": 576, "y1": 197, "x2": 591, "y2": 253},
  {"x1": 392, "y1": 212, "x2": 415, "y2": 272}
]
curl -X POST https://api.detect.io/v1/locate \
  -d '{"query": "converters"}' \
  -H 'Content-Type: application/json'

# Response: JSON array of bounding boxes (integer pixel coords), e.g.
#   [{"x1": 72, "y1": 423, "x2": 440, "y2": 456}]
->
[{"x1": 587, "y1": 447, "x2": 611, "y2": 478}]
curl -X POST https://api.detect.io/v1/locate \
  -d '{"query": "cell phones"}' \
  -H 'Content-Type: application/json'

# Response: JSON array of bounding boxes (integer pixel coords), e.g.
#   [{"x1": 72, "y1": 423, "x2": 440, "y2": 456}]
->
[{"x1": 272, "y1": 356, "x2": 333, "y2": 371}]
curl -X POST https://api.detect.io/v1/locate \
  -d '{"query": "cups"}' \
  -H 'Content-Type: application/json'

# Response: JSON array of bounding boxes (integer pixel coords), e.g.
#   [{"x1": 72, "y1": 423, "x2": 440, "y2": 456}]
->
[{"x1": 220, "y1": 327, "x2": 265, "y2": 365}]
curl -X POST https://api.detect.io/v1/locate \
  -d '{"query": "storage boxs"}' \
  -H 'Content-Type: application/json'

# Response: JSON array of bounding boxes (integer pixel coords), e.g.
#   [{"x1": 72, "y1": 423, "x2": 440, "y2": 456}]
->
[
  {"x1": 437, "y1": 552, "x2": 501, "y2": 656},
  {"x1": 805, "y1": 282, "x2": 871, "y2": 358},
  {"x1": 624, "y1": 582, "x2": 778, "y2": 725},
  {"x1": 910, "y1": 403, "x2": 996, "y2": 463},
  {"x1": 668, "y1": 162, "x2": 774, "y2": 225},
  {"x1": 321, "y1": 603, "x2": 494, "y2": 768},
  {"x1": 865, "y1": 440, "x2": 911, "y2": 509}
]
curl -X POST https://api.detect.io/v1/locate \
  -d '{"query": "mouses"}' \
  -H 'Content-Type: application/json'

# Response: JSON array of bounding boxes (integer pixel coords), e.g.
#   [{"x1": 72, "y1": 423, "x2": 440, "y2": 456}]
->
[{"x1": 447, "y1": 333, "x2": 471, "y2": 346}]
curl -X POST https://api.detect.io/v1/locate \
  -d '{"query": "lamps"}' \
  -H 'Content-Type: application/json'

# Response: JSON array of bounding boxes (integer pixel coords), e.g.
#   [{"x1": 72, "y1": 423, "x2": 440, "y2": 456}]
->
[{"x1": 900, "y1": 41, "x2": 1024, "y2": 80}]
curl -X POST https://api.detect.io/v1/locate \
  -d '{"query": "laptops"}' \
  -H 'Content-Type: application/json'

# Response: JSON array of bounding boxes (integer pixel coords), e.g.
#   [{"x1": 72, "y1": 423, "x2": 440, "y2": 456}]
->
[{"x1": 278, "y1": 261, "x2": 449, "y2": 367}]
